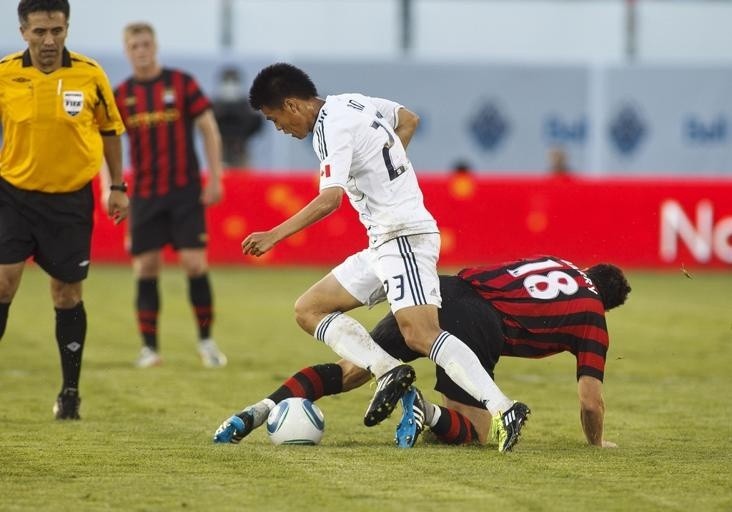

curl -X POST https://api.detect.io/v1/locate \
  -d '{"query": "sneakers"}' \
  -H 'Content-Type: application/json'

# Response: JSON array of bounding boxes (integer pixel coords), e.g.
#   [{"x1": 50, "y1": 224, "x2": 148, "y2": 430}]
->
[
  {"x1": 197, "y1": 339, "x2": 227, "y2": 368},
  {"x1": 136, "y1": 346, "x2": 161, "y2": 369},
  {"x1": 53, "y1": 384, "x2": 80, "y2": 420},
  {"x1": 365, "y1": 365, "x2": 414, "y2": 426},
  {"x1": 394, "y1": 386, "x2": 426, "y2": 446},
  {"x1": 214, "y1": 411, "x2": 254, "y2": 443},
  {"x1": 486, "y1": 399, "x2": 530, "y2": 454}
]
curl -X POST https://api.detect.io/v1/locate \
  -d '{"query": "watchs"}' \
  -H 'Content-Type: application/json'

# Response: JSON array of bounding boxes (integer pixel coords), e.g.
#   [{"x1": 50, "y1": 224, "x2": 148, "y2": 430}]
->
[{"x1": 109, "y1": 182, "x2": 130, "y2": 193}]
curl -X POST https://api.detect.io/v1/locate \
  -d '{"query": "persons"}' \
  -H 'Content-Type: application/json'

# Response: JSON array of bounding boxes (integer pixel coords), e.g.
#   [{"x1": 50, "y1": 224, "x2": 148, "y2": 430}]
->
[
  {"x1": 212, "y1": 255, "x2": 632, "y2": 449},
  {"x1": 110, "y1": 21, "x2": 228, "y2": 369},
  {"x1": 206, "y1": 68, "x2": 265, "y2": 169},
  {"x1": 0, "y1": 0, "x2": 131, "y2": 420},
  {"x1": 241, "y1": 62, "x2": 531, "y2": 454}
]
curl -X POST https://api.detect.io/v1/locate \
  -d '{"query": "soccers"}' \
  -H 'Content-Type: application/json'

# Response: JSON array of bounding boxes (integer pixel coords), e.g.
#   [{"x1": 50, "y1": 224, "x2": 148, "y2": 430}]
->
[{"x1": 266, "y1": 397, "x2": 326, "y2": 444}]
